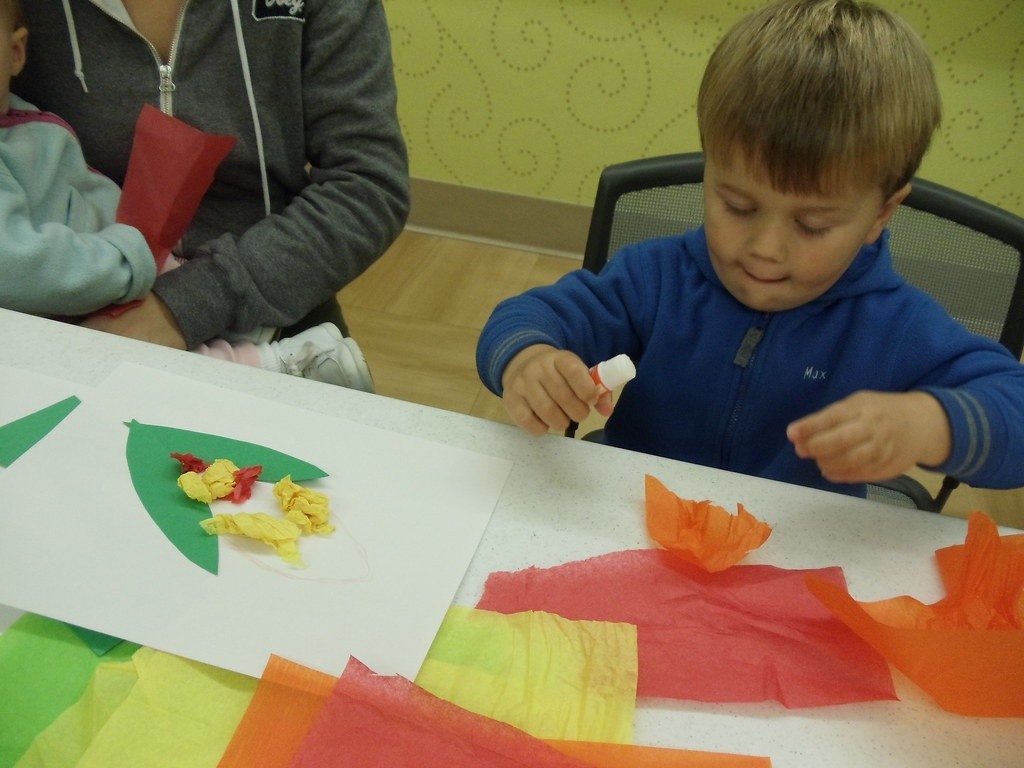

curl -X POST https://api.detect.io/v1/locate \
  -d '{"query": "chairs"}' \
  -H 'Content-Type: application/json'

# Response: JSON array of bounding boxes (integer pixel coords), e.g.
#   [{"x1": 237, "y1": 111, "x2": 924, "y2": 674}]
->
[{"x1": 564, "y1": 152, "x2": 1024, "y2": 516}]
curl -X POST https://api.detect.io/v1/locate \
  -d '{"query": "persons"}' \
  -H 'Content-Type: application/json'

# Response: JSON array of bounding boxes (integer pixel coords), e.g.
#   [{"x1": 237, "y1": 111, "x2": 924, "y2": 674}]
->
[
  {"x1": 475, "y1": 0, "x2": 1024, "y2": 499},
  {"x1": 0, "y1": 0, "x2": 377, "y2": 392},
  {"x1": 10, "y1": 0, "x2": 410, "y2": 351}
]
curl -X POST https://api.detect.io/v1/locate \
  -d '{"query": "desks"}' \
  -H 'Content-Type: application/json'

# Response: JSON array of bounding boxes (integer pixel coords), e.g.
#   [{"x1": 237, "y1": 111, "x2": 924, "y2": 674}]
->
[{"x1": 0, "y1": 303, "x2": 1024, "y2": 768}]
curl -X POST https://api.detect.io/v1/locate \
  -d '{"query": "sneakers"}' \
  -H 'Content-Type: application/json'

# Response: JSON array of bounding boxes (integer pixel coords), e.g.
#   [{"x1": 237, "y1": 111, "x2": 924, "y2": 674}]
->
[{"x1": 278, "y1": 321, "x2": 374, "y2": 392}]
